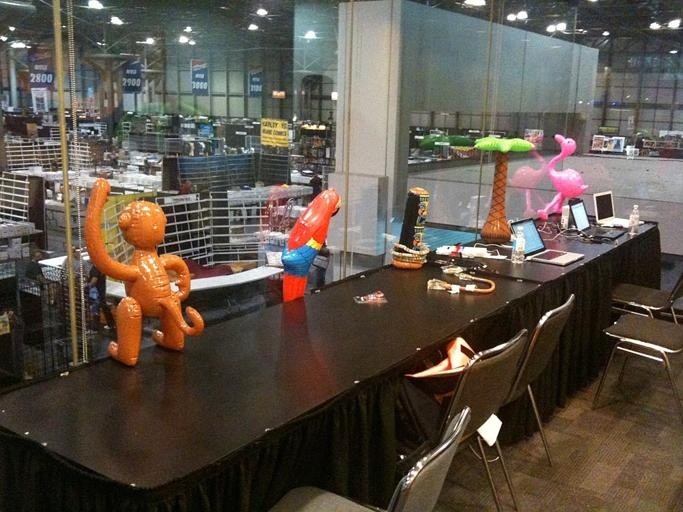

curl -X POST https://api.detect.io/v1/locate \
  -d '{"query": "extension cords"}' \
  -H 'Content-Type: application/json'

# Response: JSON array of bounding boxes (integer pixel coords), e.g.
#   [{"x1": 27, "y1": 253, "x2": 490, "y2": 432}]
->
[{"x1": 439, "y1": 244, "x2": 487, "y2": 258}]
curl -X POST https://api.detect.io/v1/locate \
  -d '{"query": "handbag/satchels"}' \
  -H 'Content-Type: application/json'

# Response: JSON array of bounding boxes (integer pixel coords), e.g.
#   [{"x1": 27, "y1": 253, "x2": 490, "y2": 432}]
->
[{"x1": 399, "y1": 337, "x2": 482, "y2": 440}]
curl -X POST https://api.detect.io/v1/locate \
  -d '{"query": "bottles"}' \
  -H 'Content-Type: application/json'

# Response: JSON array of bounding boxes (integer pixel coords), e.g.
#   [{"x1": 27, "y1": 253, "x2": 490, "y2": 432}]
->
[
  {"x1": 510, "y1": 226, "x2": 525, "y2": 263},
  {"x1": 627, "y1": 204, "x2": 640, "y2": 235}
]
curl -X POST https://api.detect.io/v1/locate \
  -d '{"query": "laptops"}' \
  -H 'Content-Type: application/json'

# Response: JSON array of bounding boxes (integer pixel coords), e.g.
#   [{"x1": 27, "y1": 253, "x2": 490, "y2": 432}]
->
[
  {"x1": 569, "y1": 200, "x2": 628, "y2": 240},
  {"x1": 510, "y1": 217, "x2": 585, "y2": 266},
  {"x1": 593, "y1": 191, "x2": 644, "y2": 228}
]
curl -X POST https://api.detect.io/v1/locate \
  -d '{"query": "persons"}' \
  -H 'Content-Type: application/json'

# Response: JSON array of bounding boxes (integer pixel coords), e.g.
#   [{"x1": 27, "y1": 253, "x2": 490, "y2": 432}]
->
[
  {"x1": 25, "y1": 249, "x2": 45, "y2": 281},
  {"x1": 178, "y1": 177, "x2": 192, "y2": 195},
  {"x1": 54, "y1": 246, "x2": 87, "y2": 326},
  {"x1": 83, "y1": 265, "x2": 116, "y2": 335}
]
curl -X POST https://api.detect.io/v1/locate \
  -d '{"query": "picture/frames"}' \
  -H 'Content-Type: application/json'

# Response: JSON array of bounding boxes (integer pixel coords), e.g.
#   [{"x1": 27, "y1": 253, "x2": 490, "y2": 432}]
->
[{"x1": 590, "y1": 135, "x2": 625, "y2": 153}]
